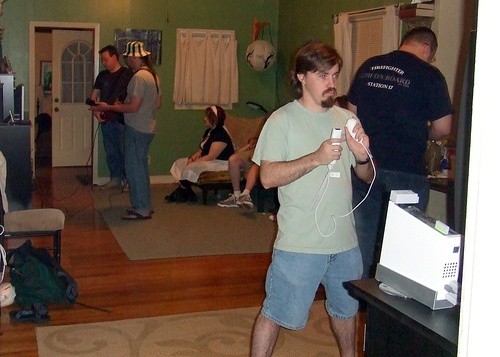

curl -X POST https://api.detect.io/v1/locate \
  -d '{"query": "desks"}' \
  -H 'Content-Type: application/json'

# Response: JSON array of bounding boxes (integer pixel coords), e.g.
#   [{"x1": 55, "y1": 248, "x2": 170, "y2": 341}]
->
[{"x1": 345, "y1": 274, "x2": 463, "y2": 355}]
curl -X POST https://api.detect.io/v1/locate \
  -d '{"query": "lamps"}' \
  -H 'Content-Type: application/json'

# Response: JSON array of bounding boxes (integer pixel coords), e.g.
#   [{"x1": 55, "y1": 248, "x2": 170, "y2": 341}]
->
[{"x1": 246, "y1": 101, "x2": 268, "y2": 116}]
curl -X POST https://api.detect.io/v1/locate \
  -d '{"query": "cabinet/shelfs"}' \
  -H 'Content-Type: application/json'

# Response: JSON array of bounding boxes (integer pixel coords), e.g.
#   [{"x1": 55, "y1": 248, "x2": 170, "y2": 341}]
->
[{"x1": 0, "y1": 121, "x2": 34, "y2": 209}]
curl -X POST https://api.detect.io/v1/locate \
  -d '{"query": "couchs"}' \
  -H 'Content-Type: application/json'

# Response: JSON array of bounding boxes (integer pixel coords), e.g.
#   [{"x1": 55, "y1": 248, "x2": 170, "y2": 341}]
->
[{"x1": 187, "y1": 110, "x2": 269, "y2": 203}]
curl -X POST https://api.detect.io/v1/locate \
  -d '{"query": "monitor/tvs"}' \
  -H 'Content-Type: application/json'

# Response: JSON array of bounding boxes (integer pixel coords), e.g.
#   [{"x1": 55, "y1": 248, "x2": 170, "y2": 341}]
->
[{"x1": 15, "y1": 83, "x2": 25, "y2": 120}]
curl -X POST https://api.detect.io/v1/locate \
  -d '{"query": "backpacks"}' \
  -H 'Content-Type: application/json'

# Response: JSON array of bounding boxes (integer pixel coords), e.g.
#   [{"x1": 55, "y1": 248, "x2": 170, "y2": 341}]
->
[{"x1": 6, "y1": 238, "x2": 77, "y2": 326}]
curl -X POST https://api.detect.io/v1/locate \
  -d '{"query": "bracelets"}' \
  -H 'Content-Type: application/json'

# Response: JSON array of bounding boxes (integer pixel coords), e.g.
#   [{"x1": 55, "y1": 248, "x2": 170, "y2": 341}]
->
[{"x1": 355, "y1": 157, "x2": 370, "y2": 164}]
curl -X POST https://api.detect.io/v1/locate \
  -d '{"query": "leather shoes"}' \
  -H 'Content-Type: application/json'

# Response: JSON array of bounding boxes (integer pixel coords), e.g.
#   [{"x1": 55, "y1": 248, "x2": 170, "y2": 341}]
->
[
  {"x1": 165, "y1": 187, "x2": 185, "y2": 201},
  {"x1": 178, "y1": 190, "x2": 198, "y2": 202}
]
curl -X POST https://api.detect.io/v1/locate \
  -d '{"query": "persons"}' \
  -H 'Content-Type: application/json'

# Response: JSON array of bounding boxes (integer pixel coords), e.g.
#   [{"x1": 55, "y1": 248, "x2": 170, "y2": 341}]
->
[
  {"x1": 163, "y1": 105, "x2": 233, "y2": 202},
  {"x1": 88, "y1": 41, "x2": 163, "y2": 220},
  {"x1": 250, "y1": 43, "x2": 377, "y2": 357},
  {"x1": 91, "y1": 45, "x2": 134, "y2": 191},
  {"x1": 217, "y1": 137, "x2": 260, "y2": 210},
  {"x1": 347, "y1": 26, "x2": 455, "y2": 356},
  {"x1": 334, "y1": 94, "x2": 348, "y2": 109}
]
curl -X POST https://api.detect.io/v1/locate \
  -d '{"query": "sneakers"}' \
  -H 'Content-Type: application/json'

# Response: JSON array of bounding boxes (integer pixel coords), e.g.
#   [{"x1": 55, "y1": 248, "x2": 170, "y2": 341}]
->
[
  {"x1": 237, "y1": 192, "x2": 254, "y2": 209},
  {"x1": 100, "y1": 181, "x2": 121, "y2": 189},
  {"x1": 121, "y1": 179, "x2": 129, "y2": 193},
  {"x1": 216, "y1": 192, "x2": 240, "y2": 207}
]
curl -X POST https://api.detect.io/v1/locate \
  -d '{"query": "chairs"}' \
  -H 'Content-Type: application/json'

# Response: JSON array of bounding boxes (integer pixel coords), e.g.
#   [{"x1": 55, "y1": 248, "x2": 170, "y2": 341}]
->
[{"x1": 0, "y1": 152, "x2": 66, "y2": 271}]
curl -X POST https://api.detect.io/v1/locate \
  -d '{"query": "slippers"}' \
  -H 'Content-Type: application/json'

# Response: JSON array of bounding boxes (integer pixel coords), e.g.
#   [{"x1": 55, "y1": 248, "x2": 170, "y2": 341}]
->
[
  {"x1": 121, "y1": 211, "x2": 155, "y2": 220},
  {"x1": 126, "y1": 207, "x2": 154, "y2": 214}
]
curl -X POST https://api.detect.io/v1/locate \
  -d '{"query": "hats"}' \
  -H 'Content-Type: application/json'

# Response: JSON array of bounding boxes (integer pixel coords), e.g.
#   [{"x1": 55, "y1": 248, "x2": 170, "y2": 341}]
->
[{"x1": 119, "y1": 40, "x2": 151, "y2": 57}]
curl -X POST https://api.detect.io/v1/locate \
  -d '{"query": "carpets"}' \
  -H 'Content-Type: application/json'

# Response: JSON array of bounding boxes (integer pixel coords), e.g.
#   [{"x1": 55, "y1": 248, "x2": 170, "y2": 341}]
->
[
  {"x1": 91, "y1": 183, "x2": 277, "y2": 261},
  {"x1": 34, "y1": 298, "x2": 367, "y2": 355}
]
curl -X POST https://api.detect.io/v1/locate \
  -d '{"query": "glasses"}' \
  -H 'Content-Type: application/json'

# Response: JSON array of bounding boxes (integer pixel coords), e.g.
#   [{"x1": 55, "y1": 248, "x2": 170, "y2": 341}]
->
[{"x1": 425, "y1": 41, "x2": 436, "y2": 62}]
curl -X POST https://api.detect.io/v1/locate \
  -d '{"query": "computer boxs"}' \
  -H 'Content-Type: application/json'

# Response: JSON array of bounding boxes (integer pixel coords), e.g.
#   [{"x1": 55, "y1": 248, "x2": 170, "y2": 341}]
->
[{"x1": 377, "y1": 200, "x2": 461, "y2": 309}]
